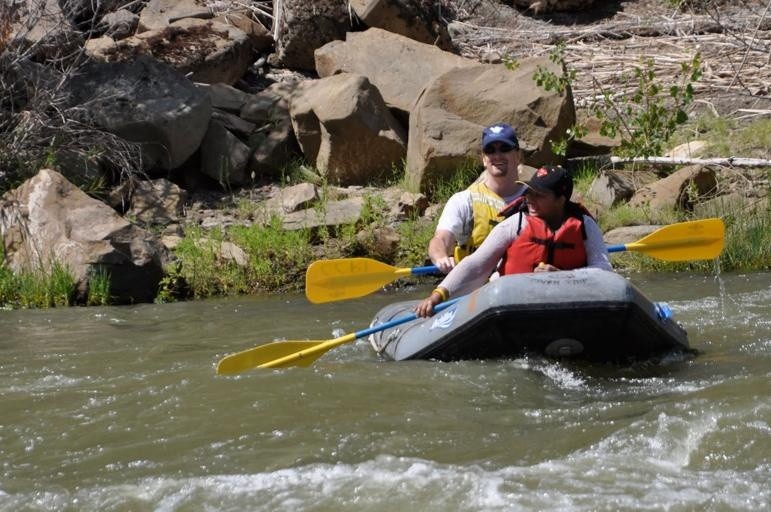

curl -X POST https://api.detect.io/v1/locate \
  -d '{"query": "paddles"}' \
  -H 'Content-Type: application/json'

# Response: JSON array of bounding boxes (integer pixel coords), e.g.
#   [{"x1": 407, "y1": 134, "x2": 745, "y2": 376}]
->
[
  {"x1": 305, "y1": 218, "x2": 724, "y2": 305},
  {"x1": 216, "y1": 296, "x2": 477, "y2": 374}
]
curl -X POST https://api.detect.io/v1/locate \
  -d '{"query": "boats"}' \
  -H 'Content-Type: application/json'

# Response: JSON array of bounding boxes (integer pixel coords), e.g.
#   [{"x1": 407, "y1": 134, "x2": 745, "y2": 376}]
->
[{"x1": 368, "y1": 266, "x2": 690, "y2": 369}]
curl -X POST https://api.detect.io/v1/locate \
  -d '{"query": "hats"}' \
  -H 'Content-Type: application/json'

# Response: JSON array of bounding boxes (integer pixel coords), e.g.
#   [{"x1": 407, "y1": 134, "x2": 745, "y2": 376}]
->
[
  {"x1": 480, "y1": 123, "x2": 518, "y2": 150},
  {"x1": 514, "y1": 164, "x2": 573, "y2": 202}
]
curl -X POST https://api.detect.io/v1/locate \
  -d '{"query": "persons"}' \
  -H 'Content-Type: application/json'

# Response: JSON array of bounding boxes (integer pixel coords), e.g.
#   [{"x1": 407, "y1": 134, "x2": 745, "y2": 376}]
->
[
  {"x1": 426, "y1": 121, "x2": 530, "y2": 280},
  {"x1": 411, "y1": 162, "x2": 614, "y2": 321}
]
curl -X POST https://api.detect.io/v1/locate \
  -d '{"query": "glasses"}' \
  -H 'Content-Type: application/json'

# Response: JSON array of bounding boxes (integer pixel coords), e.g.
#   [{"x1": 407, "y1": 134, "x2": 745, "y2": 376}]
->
[{"x1": 482, "y1": 143, "x2": 513, "y2": 154}]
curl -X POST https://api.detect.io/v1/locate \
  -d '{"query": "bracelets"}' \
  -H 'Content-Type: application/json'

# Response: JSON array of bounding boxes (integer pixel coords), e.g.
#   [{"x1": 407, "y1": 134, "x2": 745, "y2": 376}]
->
[{"x1": 431, "y1": 288, "x2": 448, "y2": 303}]
